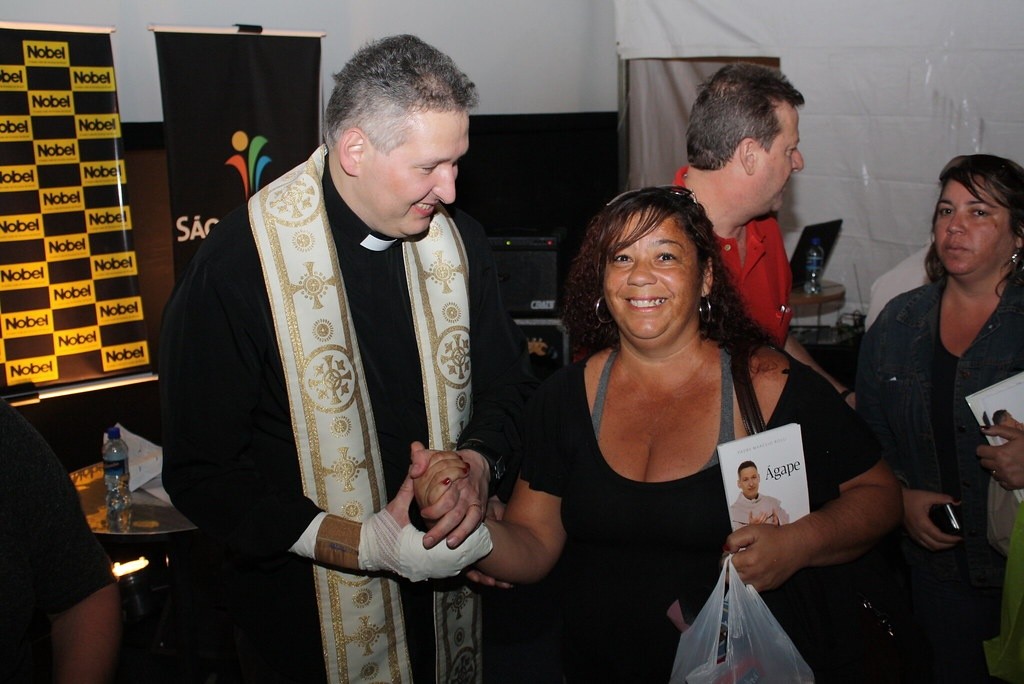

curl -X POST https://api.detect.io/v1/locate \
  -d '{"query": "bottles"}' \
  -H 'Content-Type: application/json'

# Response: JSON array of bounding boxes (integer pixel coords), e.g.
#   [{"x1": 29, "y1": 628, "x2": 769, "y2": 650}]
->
[
  {"x1": 804, "y1": 237, "x2": 824, "y2": 297},
  {"x1": 102, "y1": 427, "x2": 131, "y2": 511}
]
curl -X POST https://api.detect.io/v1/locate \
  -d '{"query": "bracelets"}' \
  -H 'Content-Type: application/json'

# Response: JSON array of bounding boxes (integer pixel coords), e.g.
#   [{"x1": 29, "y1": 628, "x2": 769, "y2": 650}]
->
[
  {"x1": 841, "y1": 389, "x2": 853, "y2": 399},
  {"x1": 459, "y1": 441, "x2": 505, "y2": 494}
]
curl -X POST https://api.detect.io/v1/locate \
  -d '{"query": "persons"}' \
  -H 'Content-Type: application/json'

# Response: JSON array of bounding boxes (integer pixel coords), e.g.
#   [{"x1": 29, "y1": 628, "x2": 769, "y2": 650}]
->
[
  {"x1": 992, "y1": 410, "x2": 1024, "y2": 446},
  {"x1": 159, "y1": 33, "x2": 532, "y2": 684},
  {"x1": 0, "y1": 401, "x2": 124, "y2": 684},
  {"x1": 730, "y1": 462, "x2": 788, "y2": 535},
  {"x1": 411, "y1": 190, "x2": 904, "y2": 683},
  {"x1": 670, "y1": 61, "x2": 857, "y2": 409},
  {"x1": 857, "y1": 155, "x2": 1024, "y2": 683}
]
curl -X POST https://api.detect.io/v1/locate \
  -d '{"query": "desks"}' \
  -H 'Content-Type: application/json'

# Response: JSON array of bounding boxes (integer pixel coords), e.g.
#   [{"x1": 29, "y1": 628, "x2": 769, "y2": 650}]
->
[
  {"x1": 68, "y1": 461, "x2": 203, "y2": 684},
  {"x1": 790, "y1": 280, "x2": 846, "y2": 305}
]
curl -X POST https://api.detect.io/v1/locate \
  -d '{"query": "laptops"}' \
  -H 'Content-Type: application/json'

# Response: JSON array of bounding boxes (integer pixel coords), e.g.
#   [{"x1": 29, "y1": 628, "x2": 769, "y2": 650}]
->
[{"x1": 790, "y1": 219, "x2": 844, "y2": 289}]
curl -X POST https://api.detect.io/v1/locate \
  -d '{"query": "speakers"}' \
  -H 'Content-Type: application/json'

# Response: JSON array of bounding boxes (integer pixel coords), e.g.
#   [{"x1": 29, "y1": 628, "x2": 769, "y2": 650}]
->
[{"x1": 484, "y1": 237, "x2": 561, "y2": 315}]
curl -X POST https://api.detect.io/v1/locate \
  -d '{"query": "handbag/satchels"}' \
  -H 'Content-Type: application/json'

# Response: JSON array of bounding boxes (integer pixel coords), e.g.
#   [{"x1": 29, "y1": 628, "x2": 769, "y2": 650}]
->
[
  {"x1": 669, "y1": 553, "x2": 815, "y2": 684},
  {"x1": 768, "y1": 550, "x2": 900, "y2": 684}
]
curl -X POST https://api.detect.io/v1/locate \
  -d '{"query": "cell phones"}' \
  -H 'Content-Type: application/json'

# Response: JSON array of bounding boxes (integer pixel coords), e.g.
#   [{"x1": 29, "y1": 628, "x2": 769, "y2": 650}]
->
[{"x1": 928, "y1": 502, "x2": 962, "y2": 536}]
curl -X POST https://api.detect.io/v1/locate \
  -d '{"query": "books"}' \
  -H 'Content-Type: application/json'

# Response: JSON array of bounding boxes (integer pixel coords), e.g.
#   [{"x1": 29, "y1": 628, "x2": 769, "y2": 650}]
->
[
  {"x1": 963, "y1": 372, "x2": 1024, "y2": 502},
  {"x1": 717, "y1": 422, "x2": 812, "y2": 554}
]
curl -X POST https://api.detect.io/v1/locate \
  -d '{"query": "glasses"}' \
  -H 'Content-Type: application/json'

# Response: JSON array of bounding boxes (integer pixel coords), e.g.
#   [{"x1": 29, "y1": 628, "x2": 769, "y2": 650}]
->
[
  {"x1": 606, "y1": 185, "x2": 700, "y2": 212},
  {"x1": 940, "y1": 155, "x2": 1024, "y2": 188}
]
